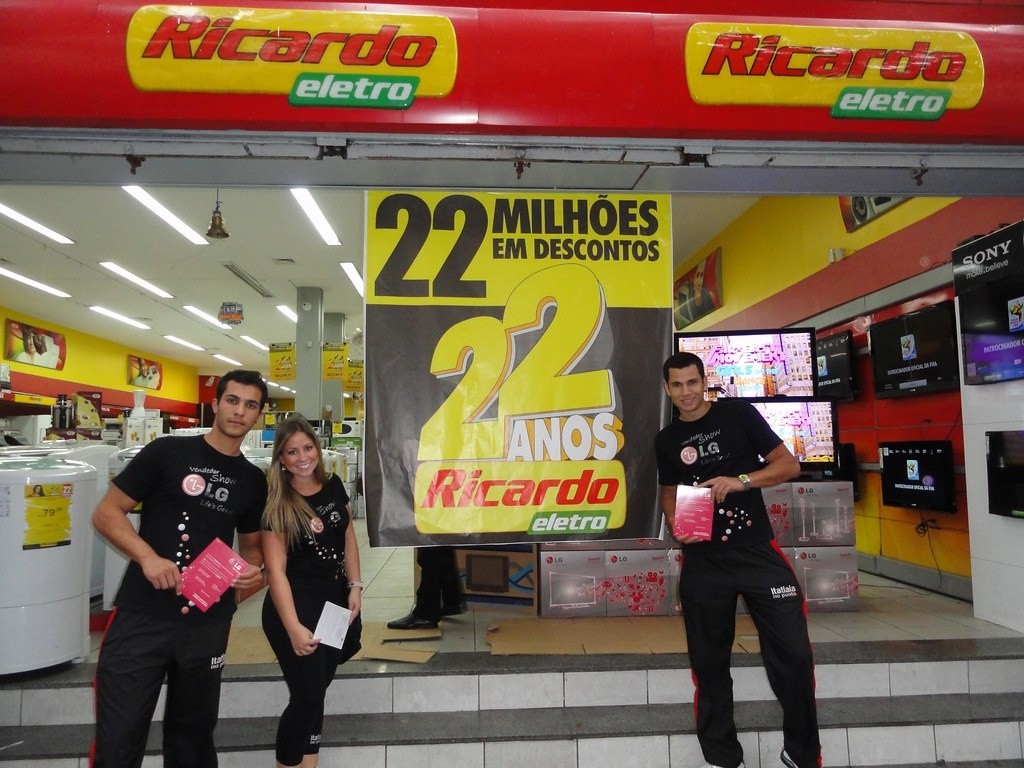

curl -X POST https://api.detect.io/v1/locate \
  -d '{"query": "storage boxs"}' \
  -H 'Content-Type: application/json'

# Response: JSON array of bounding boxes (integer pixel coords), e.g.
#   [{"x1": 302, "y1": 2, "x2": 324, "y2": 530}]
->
[{"x1": 416, "y1": 473, "x2": 859, "y2": 620}]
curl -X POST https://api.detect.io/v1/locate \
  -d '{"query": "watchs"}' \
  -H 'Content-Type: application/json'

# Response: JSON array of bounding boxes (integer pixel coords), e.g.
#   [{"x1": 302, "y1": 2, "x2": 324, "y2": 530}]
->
[
  {"x1": 736, "y1": 474, "x2": 751, "y2": 491},
  {"x1": 346, "y1": 579, "x2": 364, "y2": 592}
]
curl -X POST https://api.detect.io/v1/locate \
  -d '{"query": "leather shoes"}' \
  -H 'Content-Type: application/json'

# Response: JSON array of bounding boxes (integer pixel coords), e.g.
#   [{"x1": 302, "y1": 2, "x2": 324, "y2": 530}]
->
[
  {"x1": 439, "y1": 600, "x2": 467, "y2": 617},
  {"x1": 387, "y1": 612, "x2": 439, "y2": 630}
]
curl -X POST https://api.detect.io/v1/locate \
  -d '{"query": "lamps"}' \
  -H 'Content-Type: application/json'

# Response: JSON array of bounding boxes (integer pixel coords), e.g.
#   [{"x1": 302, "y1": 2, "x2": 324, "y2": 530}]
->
[{"x1": 208, "y1": 208, "x2": 228, "y2": 237}]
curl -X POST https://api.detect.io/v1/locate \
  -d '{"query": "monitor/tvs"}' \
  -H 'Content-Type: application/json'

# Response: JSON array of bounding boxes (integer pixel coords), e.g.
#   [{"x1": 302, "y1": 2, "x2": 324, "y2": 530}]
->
[
  {"x1": 952, "y1": 218, "x2": 1024, "y2": 387},
  {"x1": 816, "y1": 330, "x2": 856, "y2": 402},
  {"x1": 866, "y1": 299, "x2": 959, "y2": 399},
  {"x1": 985, "y1": 430, "x2": 1024, "y2": 520},
  {"x1": 751, "y1": 402, "x2": 838, "y2": 471},
  {"x1": 464, "y1": 553, "x2": 510, "y2": 595},
  {"x1": 820, "y1": 443, "x2": 859, "y2": 503},
  {"x1": 548, "y1": 571, "x2": 596, "y2": 607},
  {"x1": 876, "y1": 439, "x2": 957, "y2": 514},
  {"x1": 673, "y1": 327, "x2": 816, "y2": 401},
  {"x1": 803, "y1": 567, "x2": 851, "y2": 601}
]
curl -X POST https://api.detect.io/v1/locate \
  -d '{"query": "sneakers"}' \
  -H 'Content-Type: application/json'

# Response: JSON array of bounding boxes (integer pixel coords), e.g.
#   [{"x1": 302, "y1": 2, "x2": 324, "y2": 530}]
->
[{"x1": 781, "y1": 749, "x2": 822, "y2": 768}]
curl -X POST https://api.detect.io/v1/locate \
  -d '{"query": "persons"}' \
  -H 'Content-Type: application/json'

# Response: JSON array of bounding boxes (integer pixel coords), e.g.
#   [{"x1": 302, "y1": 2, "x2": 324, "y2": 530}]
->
[
  {"x1": 644, "y1": 352, "x2": 827, "y2": 768},
  {"x1": 386, "y1": 545, "x2": 467, "y2": 633},
  {"x1": 80, "y1": 369, "x2": 273, "y2": 768},
  {"x1": 249, "y1": 413, "x2": 367, "y2": 768}
]
